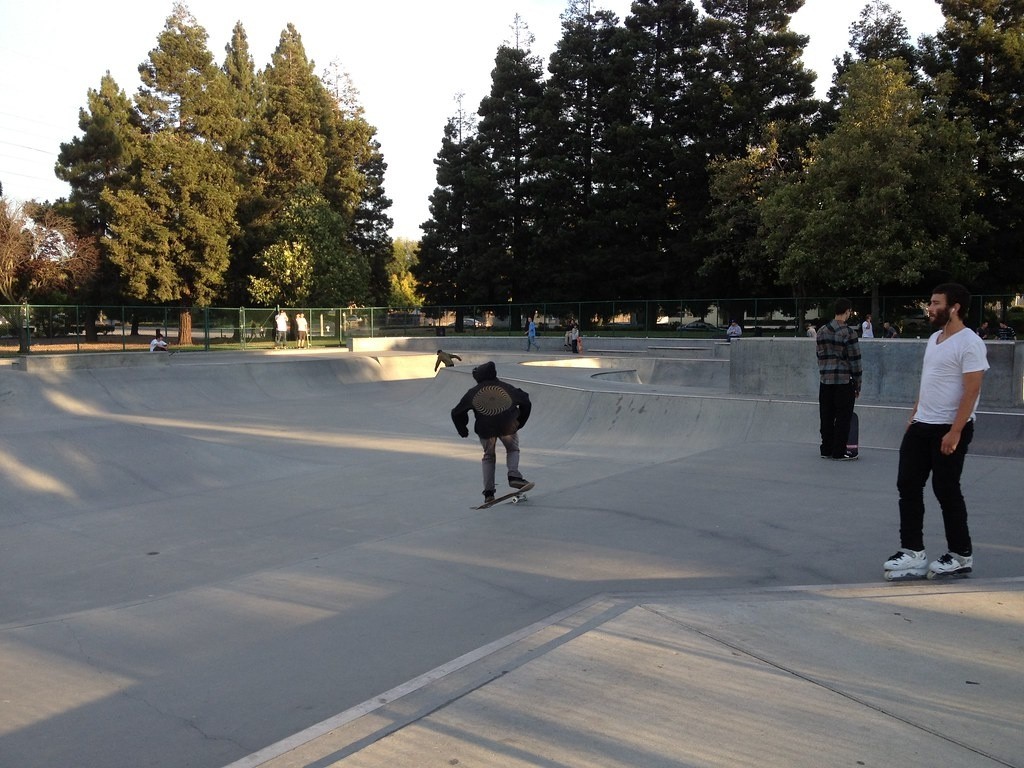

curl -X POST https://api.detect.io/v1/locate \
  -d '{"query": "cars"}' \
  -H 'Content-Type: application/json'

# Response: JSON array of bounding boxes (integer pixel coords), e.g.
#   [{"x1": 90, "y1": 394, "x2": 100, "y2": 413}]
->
[
  {"x1": 385, "y1": 313, "x2": 420, "y2": 326},
  {"x1": 676, "y1": 320, "x2": 727, "y2": 333},
  {"x1": 346, "y1": 313, "x2": 369, "y2": 322},
  {"x1": 448, "y1": 317, "x2": 484, "y2": 329}
]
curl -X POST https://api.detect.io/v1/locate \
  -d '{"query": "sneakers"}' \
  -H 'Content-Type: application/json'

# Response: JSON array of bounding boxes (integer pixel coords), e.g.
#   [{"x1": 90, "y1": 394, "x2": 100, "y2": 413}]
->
[
  {"x1": 832, "y1": 450, "x2": 858, "y2": 461},
  {"x1": 508, "y1": 475, "x2": 529, "y2": 489},
  {"x1": 482, "y1": 490, "x2": 494, "y2": 503},
  {"x1": 821, "y1": 455, "x2": 832, "y2": 458}
]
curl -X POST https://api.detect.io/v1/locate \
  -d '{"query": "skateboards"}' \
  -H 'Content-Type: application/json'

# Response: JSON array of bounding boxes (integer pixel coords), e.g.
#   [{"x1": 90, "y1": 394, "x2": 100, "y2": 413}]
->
[
  {"x1": 471, "y1": 481, "x2": 536, "y2": 511},
  {"x1": 845, "y1": 412, "x2": 861, "y2": 460},
  {"x1": 578, "y1": 338, "x2": 583, "y2": 354}
]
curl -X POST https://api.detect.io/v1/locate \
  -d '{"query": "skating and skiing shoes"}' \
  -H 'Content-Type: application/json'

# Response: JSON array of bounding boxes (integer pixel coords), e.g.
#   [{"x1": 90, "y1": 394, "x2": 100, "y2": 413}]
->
[
  {"x1": 927, "y1": 552, "x2": 973, "y2": 580},
  {"x1": 883, "y1": 548, "x2": 929, "y2": 581}
]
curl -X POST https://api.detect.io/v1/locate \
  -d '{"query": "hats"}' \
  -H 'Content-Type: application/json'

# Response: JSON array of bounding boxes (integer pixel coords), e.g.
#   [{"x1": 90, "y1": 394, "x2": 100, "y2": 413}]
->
[
  {"x1": 731, "y1": 320, "x2": 735, "y2": 323},
  {"x1": 437, "y1": 350, "x2": 442, "y2": 355}
]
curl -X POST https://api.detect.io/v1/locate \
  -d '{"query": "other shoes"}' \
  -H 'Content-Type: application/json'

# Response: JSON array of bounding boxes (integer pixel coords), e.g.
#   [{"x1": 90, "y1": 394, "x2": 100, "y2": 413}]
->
[
  {"x1": 563, "y1": 344, "x2": 567, "y2": 346},
  {"x1": 568, "y1": 344, "x2": 571, "y2": 347},
  {"x1": 537, "y1": 347, "x2": 540, "y2": 352},
  {"x1": 524, "y1": 350, "x2": 530, "y2": 352}
]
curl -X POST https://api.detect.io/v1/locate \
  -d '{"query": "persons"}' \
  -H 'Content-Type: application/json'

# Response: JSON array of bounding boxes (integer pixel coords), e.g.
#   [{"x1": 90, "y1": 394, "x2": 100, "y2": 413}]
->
[
  {"x1": 569, "y1": 325, "x2": 579, "y2": 354},
  {"x1": 259, "y1": 326, "x2": 265, "y2": 338},
  {"x1": 994, "y1": 321, "x2": 1017, "y2": 341},
  {"x1": 433, "y1": 349, "x2": 462, "y2": 372},
  {"x1": 816, "y1": 299, "x2": 862, "y2": 461},
  {"x1": 976, "y1": 321, "x2": 990, "y2": 340},
  {"x1": 727, "y1": 320, "x2": 742, "y2": 343},
  {"x1": 524, "y1": 318, "x2": 540, "y2": 352},
  {"x1": 881, "y1": 322, "x2": 897, "y2": 339},
  {"x1": 884, "y1": 283, "x2": 991, "y2": 581},
  {"x1": 450, "y1": 361, "x2": 534, "y2": 503},
  {"x1": 862, "y1": 315, "x2": 874, "y2": 338},
  {"x1": 250, "y1": 321, "x2": 256, "y2": 339},
  {"x1": 273, "y1": 309, "x2": 289, "y2": 349},
  {"x1": 296, "y1": 313, "x2": 307, "y2": 349},
  {"x1": 150, "y1": 333, "x2": 168, "y2": 351},
  {"x1": 805, "y1": 322, "x2": 817, "y2": 337},
  {"x1": 326, "y1": 325, "x2": 331, "y2": 332},
  {"x1": 564, "y1": 319, "x2": 577, "y2": 347}
]
